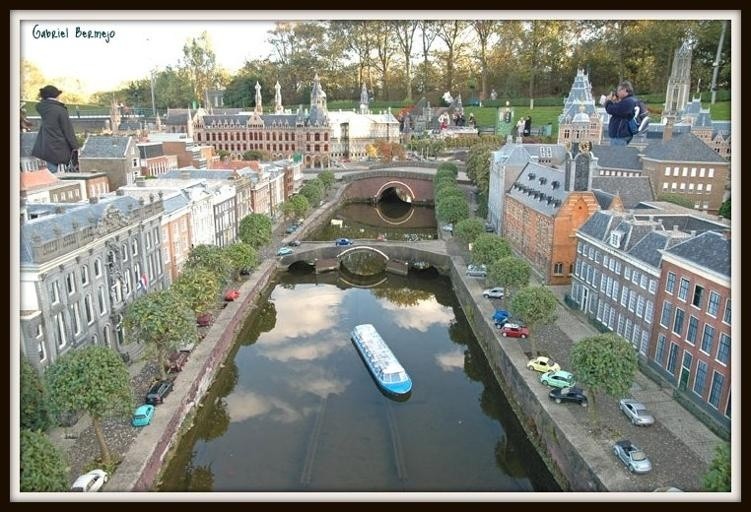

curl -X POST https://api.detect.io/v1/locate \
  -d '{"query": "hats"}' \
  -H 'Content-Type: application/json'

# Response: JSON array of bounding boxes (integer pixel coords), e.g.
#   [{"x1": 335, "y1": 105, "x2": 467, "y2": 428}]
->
[{"x1": 39, "y1": 86, "x2": 63, "y2": 98}]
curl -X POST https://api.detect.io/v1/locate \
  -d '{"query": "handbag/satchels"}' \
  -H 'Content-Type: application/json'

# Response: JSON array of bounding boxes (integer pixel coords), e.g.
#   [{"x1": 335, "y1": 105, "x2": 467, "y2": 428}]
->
[
  {"x1": 629, "y1": 99, "x2": 651, "y2": 137},
  {"x1": 67, "y1": 160, "x2": 81, "y2": 174}
]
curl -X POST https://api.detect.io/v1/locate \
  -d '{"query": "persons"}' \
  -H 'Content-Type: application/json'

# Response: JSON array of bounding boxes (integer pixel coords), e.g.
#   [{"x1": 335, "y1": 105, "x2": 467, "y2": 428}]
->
[
  {"x1": 437, "y1": 106, "x2": 476, "y2": 130},
  {"x1": 30, "y1": 85, "x2": 81, "y2": 174},
  {"x1": 20, "y1": 102, "x2": 32, "y2": 133},
  {"x1": 603, "y1": 79, "x2": 638, "y2": 146},
  {"x1": 511, "y1": 114, "x2": 532, "y2": 137},
  {"x1": 397, "y1": 111, "x2": 415, "y2": 133}
]
